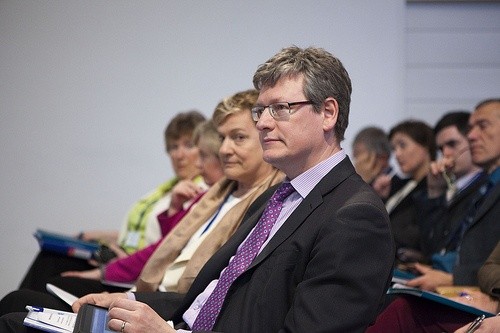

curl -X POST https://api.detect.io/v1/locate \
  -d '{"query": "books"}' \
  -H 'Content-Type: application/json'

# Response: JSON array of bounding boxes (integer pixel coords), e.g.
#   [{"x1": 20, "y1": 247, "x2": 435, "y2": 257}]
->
[{"x1": 391, "y1": 268, "x2": 421, "y2": 284}]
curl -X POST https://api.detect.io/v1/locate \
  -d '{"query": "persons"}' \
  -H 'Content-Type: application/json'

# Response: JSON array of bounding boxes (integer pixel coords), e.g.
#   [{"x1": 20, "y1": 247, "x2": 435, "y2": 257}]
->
[
  {"x1": 21, "y1": 111, "x2": 206, "y2": 292},
  {"x1": 385, "y1": 99, "x2": 500, "y2": 307},
  {"x1": 372, "y1": 120, "x2": 436, "y2": 250},
  {"x1": 360, "y1": 239, "x2": 499, "y2": 332},
  {"x1": 392, "y1": 112, "x2": 493, "y2": 267},
  {"x1": 37, "y1": 120, "x2": 224, "y2": 297},
  {"x1": 0, "y1": 45, "x2": 393, "y2": 333},
  {"x1": 354, "y1": 126, "x2": 401, "y2": 189},
  {"x1": 34, "y1": 88, "x2": 292, "y2": 308}
]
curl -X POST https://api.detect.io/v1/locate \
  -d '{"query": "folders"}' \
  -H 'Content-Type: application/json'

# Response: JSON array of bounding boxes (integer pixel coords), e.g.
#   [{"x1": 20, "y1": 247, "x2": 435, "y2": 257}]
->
[{"x1": 32, "y1": 229, "x2": 106, "y2": 259}]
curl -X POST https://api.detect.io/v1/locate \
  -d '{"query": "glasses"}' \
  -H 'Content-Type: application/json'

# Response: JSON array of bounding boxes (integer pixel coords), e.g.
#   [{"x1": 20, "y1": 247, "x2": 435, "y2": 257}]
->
[{"x1": 251, "y1": 100, "x2": 313, "y2": 122}]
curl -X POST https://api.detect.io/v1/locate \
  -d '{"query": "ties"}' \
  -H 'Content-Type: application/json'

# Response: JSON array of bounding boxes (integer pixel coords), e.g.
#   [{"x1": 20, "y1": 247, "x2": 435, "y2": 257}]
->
[{"x1": 192, "y1": 183, "x2": 296, "y2": 333}]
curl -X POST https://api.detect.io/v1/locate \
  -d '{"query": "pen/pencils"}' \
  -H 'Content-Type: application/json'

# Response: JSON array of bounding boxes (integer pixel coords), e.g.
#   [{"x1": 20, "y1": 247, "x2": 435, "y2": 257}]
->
[{"x1": 464, "y1": 312, "x2": 487, "y2": 333}]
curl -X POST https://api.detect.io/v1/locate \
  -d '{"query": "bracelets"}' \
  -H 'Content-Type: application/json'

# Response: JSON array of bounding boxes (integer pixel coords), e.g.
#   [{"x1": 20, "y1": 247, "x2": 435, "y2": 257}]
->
[{"x1": 98, "y1": 263, "x2": 105, "y2": 282}]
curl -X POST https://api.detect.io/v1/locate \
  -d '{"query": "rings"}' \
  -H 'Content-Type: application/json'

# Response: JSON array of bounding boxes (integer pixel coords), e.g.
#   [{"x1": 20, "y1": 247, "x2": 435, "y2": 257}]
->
[{"x1": 120, "y1": 320, "x2": 127, "y2": 332}]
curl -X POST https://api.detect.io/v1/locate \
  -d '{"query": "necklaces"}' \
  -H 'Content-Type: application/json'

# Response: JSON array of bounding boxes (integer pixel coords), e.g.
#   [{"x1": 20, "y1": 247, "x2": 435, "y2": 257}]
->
[{"x1": 200, "y1": 186, "x2": 235, "y2": 236}]
